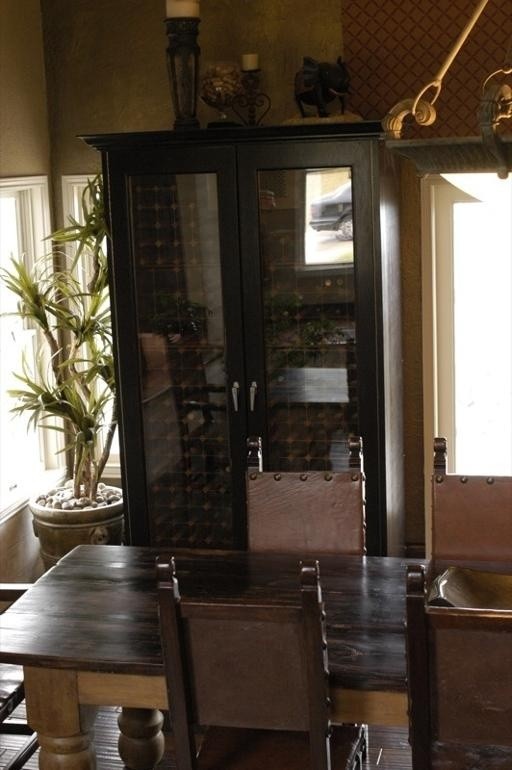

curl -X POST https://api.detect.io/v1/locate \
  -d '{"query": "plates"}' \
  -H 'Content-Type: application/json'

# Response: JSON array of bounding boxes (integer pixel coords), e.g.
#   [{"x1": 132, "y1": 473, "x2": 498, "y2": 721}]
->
[{"x1": 77, "y1": 123, "x2": 405, "y2": 557}]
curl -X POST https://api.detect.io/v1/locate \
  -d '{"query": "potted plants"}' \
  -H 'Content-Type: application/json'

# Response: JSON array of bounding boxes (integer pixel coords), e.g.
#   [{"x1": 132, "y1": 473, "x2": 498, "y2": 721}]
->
[{"x1": 8, "y1": 173, "x2": 125, "y2": 575}]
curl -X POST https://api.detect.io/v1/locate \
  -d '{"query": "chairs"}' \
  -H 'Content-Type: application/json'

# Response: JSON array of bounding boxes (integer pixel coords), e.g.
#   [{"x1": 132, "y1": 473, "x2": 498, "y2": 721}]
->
[
  {"x1": 0, "y1": 433, "x2": 511, "y2": 769},
  {"x1": 159, "y1": 296, "x2": 230, "y2": 491}
]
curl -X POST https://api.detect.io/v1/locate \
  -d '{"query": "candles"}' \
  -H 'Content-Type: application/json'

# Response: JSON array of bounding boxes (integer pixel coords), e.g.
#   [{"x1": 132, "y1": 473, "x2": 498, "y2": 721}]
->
[
  {"x1": 165, "y1": 0, "x2": 200, "y2": 19},
  {"x1": 242, "y1": 53, "x2": 259, "y2": 70}
]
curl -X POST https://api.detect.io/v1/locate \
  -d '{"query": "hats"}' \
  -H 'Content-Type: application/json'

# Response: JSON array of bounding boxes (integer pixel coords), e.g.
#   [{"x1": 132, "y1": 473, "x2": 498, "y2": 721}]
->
[{"x1": 309, "y1": 180, "x2": 353, "y2": 241}]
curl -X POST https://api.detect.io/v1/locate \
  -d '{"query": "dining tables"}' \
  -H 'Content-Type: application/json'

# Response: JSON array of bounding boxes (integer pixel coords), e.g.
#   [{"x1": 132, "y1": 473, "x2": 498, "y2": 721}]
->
[{"x1": 8, "y1": 173, "x2": 125, "y2": 575}]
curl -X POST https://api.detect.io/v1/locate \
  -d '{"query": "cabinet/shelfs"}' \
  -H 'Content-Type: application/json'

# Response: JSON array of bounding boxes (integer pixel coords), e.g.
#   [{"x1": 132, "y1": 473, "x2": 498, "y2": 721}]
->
[{"x1": 77, "y1": 123, "x2": 405, "y2": 557}]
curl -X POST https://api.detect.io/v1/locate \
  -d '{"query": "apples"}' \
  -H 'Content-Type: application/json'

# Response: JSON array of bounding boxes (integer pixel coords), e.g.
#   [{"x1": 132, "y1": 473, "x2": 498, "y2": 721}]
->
[
  {"x1": 242, "y1": 53, "x2": 259, "y2": 70},
  {"x1": 165, "y1": 0, "x2": 200, "y2": 19}
]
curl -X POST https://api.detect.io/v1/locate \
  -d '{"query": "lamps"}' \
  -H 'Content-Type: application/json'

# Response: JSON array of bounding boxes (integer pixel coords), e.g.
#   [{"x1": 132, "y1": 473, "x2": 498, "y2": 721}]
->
[{"x1": 159, "y1": 296, "x2": 230, "y2": 491}]
